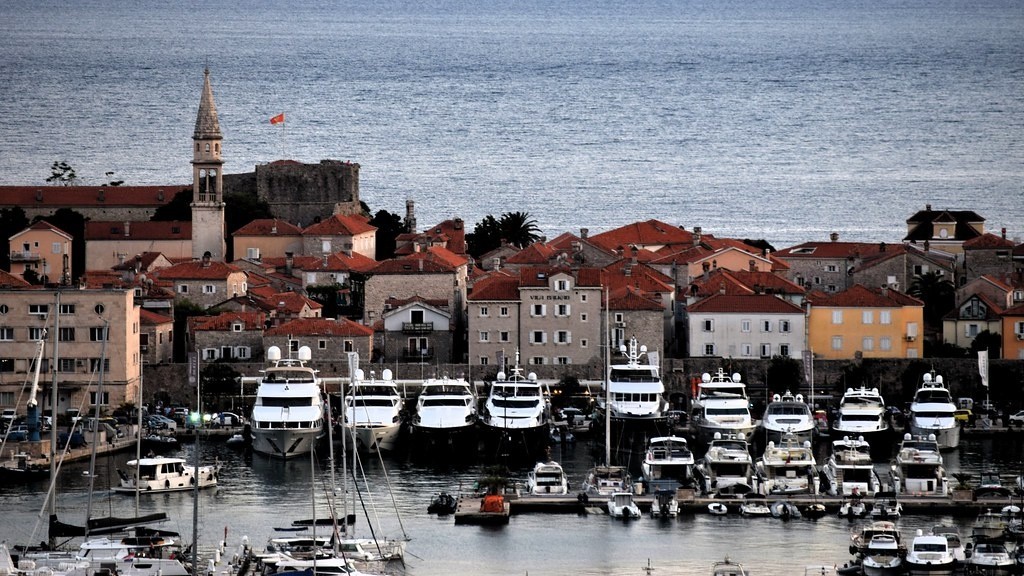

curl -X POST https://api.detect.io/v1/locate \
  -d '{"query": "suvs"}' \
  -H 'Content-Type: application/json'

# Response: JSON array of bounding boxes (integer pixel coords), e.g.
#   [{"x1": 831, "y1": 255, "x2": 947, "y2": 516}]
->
[{"x1": 65, "y1": 408, "x2": 81, "y2": 422}]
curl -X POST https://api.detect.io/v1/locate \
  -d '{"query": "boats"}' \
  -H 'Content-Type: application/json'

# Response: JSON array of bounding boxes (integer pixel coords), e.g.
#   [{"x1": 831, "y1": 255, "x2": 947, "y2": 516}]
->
[
  {"x1": 820, "y1": 436, "x2": 883, "y2": 496},
  {"x1": 548, "y1": 400, "x2": 594, "y2": 445},
  {"x1": 849, "y1": 521, "x2": 907, "y2": 575},
  {"x1": 906, "y1": 529, "x2": 956, "y2": 575},
  {"x1": 408, "y1": 326, "x2": 480, "y2": 452},
  {"x1": 934, "y1": 506, "x2": 1024, "y2": 576},
  {"x1": 838, "y1": 494, "x2": 868, "y2": 518},
  {"x1": 580, "y1": 287, "x2": 634, "y2": 494},
  {"x1": 973, "y1": 469, "x2": 1011, "y2": 499},
  {"x1": 890, "y1": 433, "x2": 952, "y2": 496},
  {"x1": 802, "y1": 563, "x2": 839, "y2": 576},
  {"x1": 740, "y1": 494, "x2": 771, "y2": 520},
  {"x1": 772, "y1": 499, "x2": 801, "y2": 520},
  {"x1": 649, "y1": 487, "x2": 681, "y2": 519},
  {"x1": 479, "y1": 344, "x2": 553, "y2": 453},
  {"x1": 336, "y1": 326, "x2": 409, "y2": 455},
  {"x1": 755, "y1": 424, "x2": 821, "y2": 495},
  {"x1": 596, "y1": 334, "x2": 671, "y2": 441},
  {"x1": 141, "y1": 433, "x2": 179, "y2": 447},
  {"x1": 762, "y1": 388, "x2": 814, "y2": 448},
  {"x1": 909, "y1": 363, "x2": 961, "y2": 450},
  {"x1": 690, "y1": 367, "x2": 762, "y2": 446},
  {"x1": 710, "y1": 556, "x2": 749, "y2": 576},
  {"x1": 607, "y1": 489, "x2": 642, "y2": 520},
  {"x1": 693, "y1": 432, "x2": 756, "y2": 492},
  {"x1": 642, "y1": 435, "x2": 697, "y2": 491},
  {"x1": 871, "y1": 492, "x2": 903, "y2": 519},
  {"x1": 525, "y1": 445, "x2": 570, "y2": 497},
  {"x1": 252, "y1": 351, "x2": 408, "y2": 575},
  {"x1": 251, "y1": 333, "x2": 334, "y2": 460},
  {"x1": 0, "y1": 291, "x2": 226, "y2": 576},
  {"x1": 707, "y1": 503, "x2": 727, "y2": 516},
  {"x1": 111, "y1": 449, "x2": 224, "y2": 496},
  {"x1": 427, "y1": 491, "x2": 457, "y2": 515},
  {"x1": 831, "y1": 359, "x2": 889, "y2": 442},
  {"x1": 1016, "y1": 461, "x2": 1023, "y2": 495},
  {"x1": 805, "y1": 503, "x2": 826, "y2": 519}
]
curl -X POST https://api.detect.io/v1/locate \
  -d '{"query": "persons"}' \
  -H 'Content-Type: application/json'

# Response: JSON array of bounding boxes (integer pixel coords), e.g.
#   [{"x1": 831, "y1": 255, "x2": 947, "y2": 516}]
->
[{"x1": 852, "y1": 487, "x2": 859, "y2": 500}]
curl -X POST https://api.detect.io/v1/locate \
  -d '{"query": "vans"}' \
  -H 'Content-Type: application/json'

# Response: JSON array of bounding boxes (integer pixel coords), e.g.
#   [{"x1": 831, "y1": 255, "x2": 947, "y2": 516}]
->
[{"x1": 953, "y1": 408, "x2": 973, "y2": 422}]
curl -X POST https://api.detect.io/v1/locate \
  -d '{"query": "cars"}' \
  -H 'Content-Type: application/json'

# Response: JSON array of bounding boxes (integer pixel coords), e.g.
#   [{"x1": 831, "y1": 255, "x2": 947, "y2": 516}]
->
[
  {"x1": 90, "y1": 407, "x2": 243, "y2": 431},
  {"x1": 1008, "y1": 410, "x2": 1024, "y2": 425},
  {"x1": 0, "y1": 409, "x2": 54, "y2": 440},
  {"x1": 982, "y1": 400, "x2": 996, "y2": 410}
]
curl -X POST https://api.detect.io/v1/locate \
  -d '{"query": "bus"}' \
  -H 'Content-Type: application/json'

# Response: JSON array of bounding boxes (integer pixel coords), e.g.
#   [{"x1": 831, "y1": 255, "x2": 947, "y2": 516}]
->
[{"x1": 951, "y1": 398, "x2": 973, "y2": 409}]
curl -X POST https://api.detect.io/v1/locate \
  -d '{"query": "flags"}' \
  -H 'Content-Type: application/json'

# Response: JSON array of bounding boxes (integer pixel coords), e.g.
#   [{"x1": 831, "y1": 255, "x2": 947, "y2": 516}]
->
[{"x1": 270, "y1": 114, "x2": 284, "y2": 124}]
